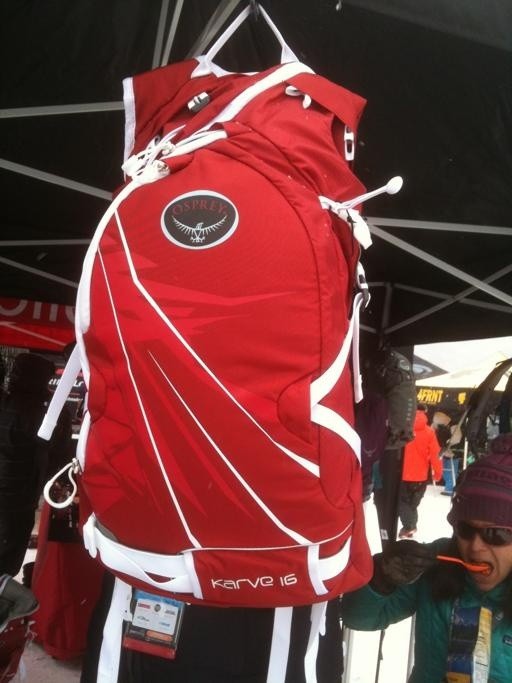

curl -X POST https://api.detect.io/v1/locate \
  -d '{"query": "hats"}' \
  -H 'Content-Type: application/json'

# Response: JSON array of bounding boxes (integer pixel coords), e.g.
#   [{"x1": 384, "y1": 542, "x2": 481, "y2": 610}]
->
[{"x1": 447, "y1": 436, "x2": 512, "y2": 527}]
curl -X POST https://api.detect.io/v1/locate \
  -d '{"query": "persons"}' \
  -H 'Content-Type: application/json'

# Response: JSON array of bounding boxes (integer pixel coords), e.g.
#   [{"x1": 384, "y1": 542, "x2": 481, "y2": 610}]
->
[
  {"x1": 0, "y1": 349, "x2": 74, "y2": 630},
  {"x1": 339, "y1": 391, "x2": 512, "y2": 683}
]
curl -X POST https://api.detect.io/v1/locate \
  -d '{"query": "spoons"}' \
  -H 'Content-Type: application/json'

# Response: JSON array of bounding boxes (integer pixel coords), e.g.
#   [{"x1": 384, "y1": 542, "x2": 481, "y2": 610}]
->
[{"x1": 434, "y1": 553, "x2": 489, "y2": 570}]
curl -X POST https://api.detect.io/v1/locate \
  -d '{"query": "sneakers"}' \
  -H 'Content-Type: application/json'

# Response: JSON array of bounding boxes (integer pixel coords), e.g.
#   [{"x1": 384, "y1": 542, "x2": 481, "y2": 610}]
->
[{"x1": 399, "y1": 527, "x2": 417, "y2": 540}]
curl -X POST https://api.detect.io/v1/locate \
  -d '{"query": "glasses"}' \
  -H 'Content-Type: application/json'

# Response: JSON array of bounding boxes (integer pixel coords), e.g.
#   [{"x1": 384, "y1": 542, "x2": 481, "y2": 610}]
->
[{"x1": 452, "y1": 525, "x2": 511, "y2": 550}]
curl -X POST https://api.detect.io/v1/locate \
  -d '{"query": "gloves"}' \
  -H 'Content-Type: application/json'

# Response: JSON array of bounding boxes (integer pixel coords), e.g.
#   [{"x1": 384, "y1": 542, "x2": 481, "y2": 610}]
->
[{"x1": 372, "y1": 540, "x2": 438, "y2": 595}]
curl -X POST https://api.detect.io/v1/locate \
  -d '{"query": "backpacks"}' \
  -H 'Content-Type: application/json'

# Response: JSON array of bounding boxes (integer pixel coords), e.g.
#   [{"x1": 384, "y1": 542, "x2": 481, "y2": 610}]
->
[{"x1": 38, "y1": 57, "x2": 404, "y2": 617}]
